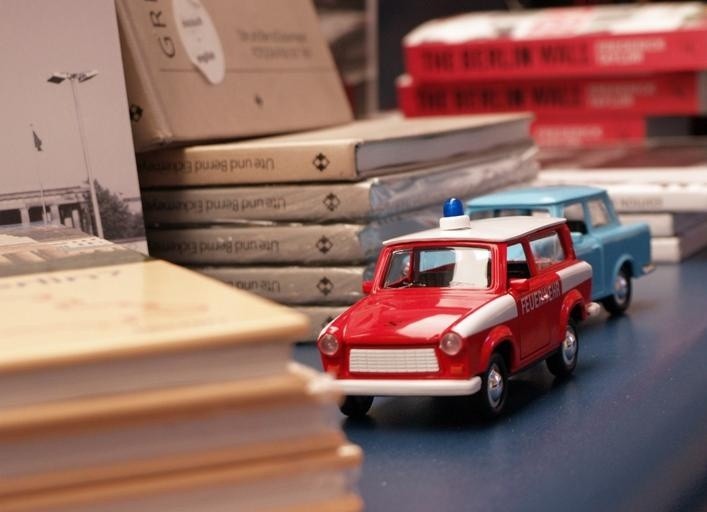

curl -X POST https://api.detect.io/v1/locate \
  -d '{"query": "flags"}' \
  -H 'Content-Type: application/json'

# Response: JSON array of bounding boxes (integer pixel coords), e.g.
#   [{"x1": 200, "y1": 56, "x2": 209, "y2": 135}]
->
[{"x1": 32, "y1": 129, "x2": 42, "y2": 151}]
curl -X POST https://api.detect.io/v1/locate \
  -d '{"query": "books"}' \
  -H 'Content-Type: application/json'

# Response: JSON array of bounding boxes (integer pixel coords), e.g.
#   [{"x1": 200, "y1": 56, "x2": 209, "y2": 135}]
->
[
  {"x1": 395, "y1": 2, "x2": 705, "y2": 265},
  {"x1": 136, "y1": 112, "x2": 536, "y2": 189},
  {"x1": 0, "y1": 360, "x2": 347, "y2": 497},
  {"x1": 115, "y1": 1, "x2": 357, "y2": 156},
  {"x1": 282, "y1": 302, "x2": 352, "y2": 344},
  {"x1": 146, "y1": 181, "x2": 538, "y2": 266},
  {"x1": 189, "y1": 255, "x2": 405, "y2": 307},
  {"x1": 139, "y1": 144, "x2": 540, "y2": 225},
  {"x1": 0, "y1": 220, "x2": 310, "y2": 430},
  {"x1": 2, "y1": 441, "x2": 368, "y2": 512}
]
